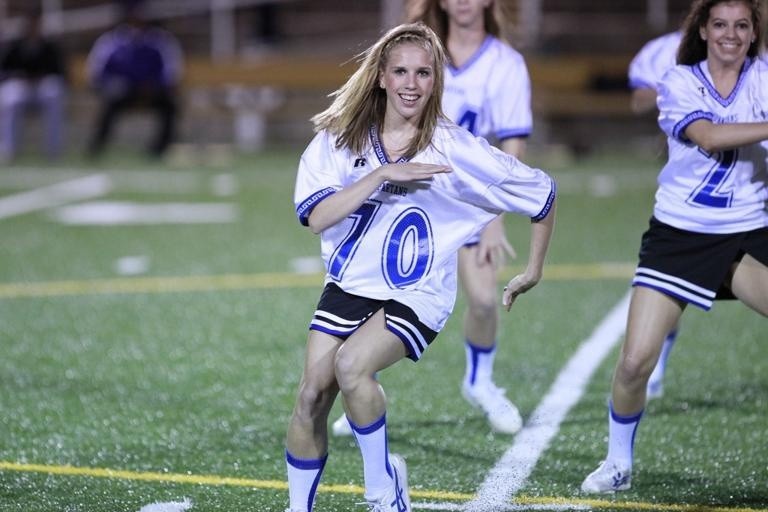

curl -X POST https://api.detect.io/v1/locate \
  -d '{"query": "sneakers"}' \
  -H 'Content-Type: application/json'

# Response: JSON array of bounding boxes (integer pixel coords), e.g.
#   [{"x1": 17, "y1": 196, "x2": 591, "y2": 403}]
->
[
  {"x1": 581, "y1": 458, "x2": 634, "y2": 493},
  {"x1": 355, "y1": 453, "x2": 412, "y2": 512},
  {"x1": 460, "y1": 376, "x2": 523, "y2": 433},
  {"x1": 646, "y1": 372, "x2": 664, "y2": 400},
  {"x1": 332, "y1": 413, "x2": 353, "y2": 436}
]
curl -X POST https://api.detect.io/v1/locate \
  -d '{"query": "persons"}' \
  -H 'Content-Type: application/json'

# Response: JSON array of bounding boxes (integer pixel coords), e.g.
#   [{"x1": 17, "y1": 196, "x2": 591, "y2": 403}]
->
[
  {"x1": 627, "y1": 1, "x2": 768, "y2": 403},
  {"x1": 82, "y1": 3, "x2": 188, "y2": 157},
  {"x1": 581, "y1": 0, "x2": 768, "y2": 497},
  {"x1": 1, "y1": 11, "x2": 69, "y2": 158},
  {"x1": 334, "y1": 1, "x2": 532, "y2": 436},
  {"x1": 286, "y1": 20, "x2": 556, "y2": 511}
]
